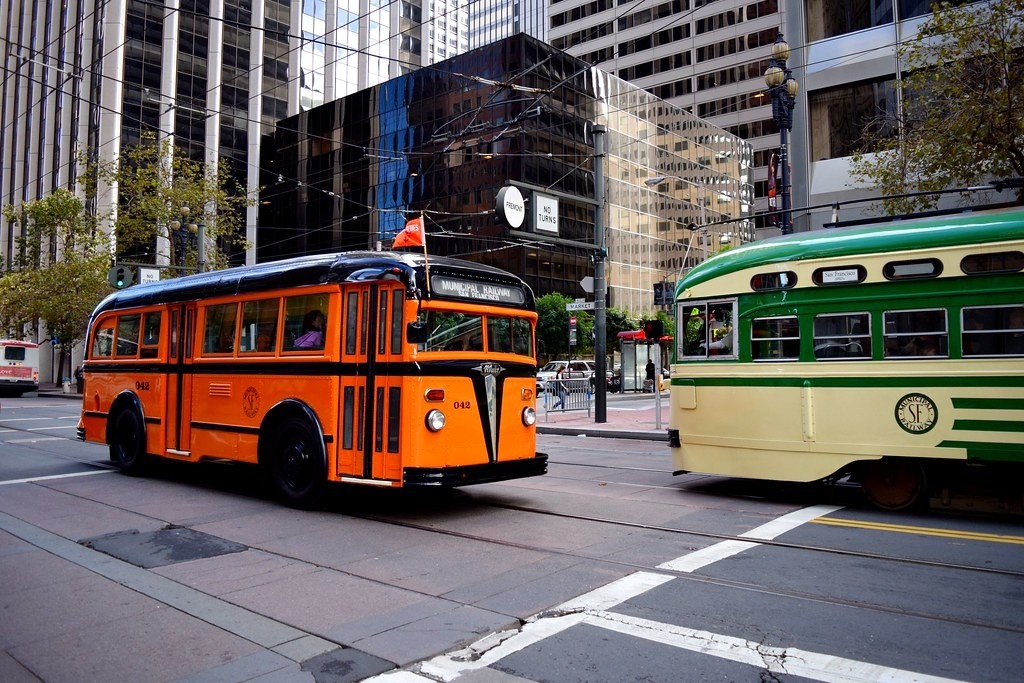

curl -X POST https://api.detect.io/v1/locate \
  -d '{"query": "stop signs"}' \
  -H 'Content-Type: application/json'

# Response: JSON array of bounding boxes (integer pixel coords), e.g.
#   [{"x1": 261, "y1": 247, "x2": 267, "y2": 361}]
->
[{"x1": 570, "y1": 317, "x2": 576, "y2": 325}]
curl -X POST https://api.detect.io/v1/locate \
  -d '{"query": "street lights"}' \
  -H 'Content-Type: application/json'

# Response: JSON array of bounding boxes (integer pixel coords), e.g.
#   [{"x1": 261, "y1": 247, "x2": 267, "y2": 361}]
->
[
  {"x1": 764, "y1": 32, "x2": 799, "y2": 236},
  {"x1": 171, "y1": 201, "x2": 198, "y2": 278},
  {"x1": 662, "y1": 266, "x2": 693, "y2": 371},
  {"x1": 643, "y1": 175, "x2": 708, "y2": 262}
]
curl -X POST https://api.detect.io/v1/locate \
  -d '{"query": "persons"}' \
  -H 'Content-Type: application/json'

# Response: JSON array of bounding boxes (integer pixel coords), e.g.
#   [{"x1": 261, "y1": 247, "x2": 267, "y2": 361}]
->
[
  {"x1": 663, "y1": 365, "x2": 670, "y2": 375},
  {"x1": 781, "y1": 315, "x2": 871, "y2": 357},
  {"x1": 141, "y1": 325, "x2": 159, "y2": 358},
  {"x1": 293, "y1": 309, "x2": 325, "y2": 349},
  {"x1": 964, "y1": 309, "x2": 1024, "y2": 354},
  {"x1": 645, "y1": 359, "x2": 655, "y2": 393},
  {"x1": 886, "y1": 312, "x2": 942, "y2": 355},
  {"x1": 257, "y1": 317, "x2": 276, "y2": 352},
  {"x1": 217, "y1": 334, "x2": 232, "y2": 352},
  {"x1": 769, "y1": 157, "x2": 775, "y2": 187},
  {"x1": 73, "y1": 366, "x2": 81, "y2": 379},
  {"x1": 700, "y1": 328, "x2": 733, "y2": 355},
  {"x1": 553, "y1": 365, "x2": 568, "y2": 409},
  {"x1": 444, "y1": 319, "x2": 483, "y2": 351}
]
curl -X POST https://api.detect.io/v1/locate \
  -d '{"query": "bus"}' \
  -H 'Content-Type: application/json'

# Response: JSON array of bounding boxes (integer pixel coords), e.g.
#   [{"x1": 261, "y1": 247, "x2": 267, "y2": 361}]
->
[
  {"x1": 75, "y1": 249, "x2": 549, "y2": 513},
  {"x1": 657, "y1": 219, "x2": 1024, "y2": 521}
]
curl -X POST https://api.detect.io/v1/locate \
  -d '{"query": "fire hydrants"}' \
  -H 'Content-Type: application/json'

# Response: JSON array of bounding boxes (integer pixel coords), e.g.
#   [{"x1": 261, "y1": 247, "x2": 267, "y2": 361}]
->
[{"x1": 61, "y1": 376, "x2": 71, "y2": 394}]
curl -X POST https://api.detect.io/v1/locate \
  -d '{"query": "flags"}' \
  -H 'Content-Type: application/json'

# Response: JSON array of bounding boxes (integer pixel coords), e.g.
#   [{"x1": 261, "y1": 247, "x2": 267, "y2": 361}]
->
[{"x1": 391, "y1": 216, "x2": 425, "y2": 248}]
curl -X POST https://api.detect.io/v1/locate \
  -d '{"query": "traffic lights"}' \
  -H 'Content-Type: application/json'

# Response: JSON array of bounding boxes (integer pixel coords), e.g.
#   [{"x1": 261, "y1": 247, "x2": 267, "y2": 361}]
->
[
  {"x1": 645, "y1": 320, "x2": 653, "y2": 340},
  {"x1": 108, "y1": 266, "x2": 133, "y2": 289}
]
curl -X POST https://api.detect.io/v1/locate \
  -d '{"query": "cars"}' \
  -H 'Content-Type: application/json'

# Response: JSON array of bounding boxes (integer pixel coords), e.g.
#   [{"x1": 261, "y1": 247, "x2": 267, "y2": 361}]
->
[{"x1": 535, "y1": 360, "x2": 621, "y2": 398}]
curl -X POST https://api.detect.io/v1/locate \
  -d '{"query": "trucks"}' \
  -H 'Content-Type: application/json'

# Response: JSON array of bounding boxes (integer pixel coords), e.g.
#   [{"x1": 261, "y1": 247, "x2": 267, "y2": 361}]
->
[{"x1": 0, "y1": 339, "x2": 39, "y2": 398}]
